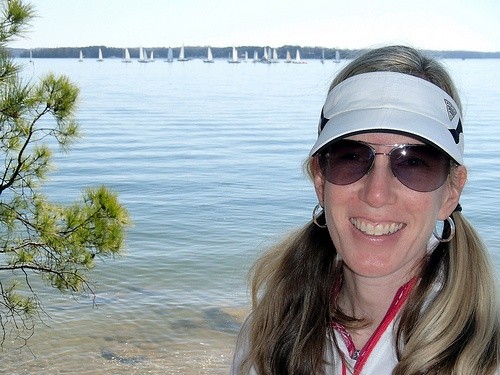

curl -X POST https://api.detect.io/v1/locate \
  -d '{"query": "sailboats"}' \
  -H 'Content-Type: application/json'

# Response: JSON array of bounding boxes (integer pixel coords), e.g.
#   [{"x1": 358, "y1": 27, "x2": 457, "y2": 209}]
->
[
  {"x1": 243, "y1": 51, "x2": 249, "y2": 63},
  {"x1": 235, "y1": 49, "x2": 238, "y2": 61},
  {"x1": 284, "y1": 50, "x2": 291, "y2": 63},
  {"x1": 96, "y1": 48, "x2": 104, "y2": 61},
  {"x1": 320, "y1": 50, "x2": 325, "y2": 64},
  {"x1": 267, "y1": 48, "x2": 272, "y2": 63},
  {"x1": 149, "y1": 52, "x2": 155, "y2": 62},
  {"x1": 177, "y1": 46, "x2": 188, "y2": 61},
  {"x1": 122, "y1": 48, "x2": 132, "y2": 63},
  {"x1": 272, "y1": 49, "x2": 280, "y2": 63},
  {"x1": 260, "y1": 47, "x2": 268, "y2": 61},
  {"x1": 29, "y1": 50, "x2": 34, "y2": 63},
  {"x1": 228, "y1": 48, "x2": 241, "y2": 63},
  {"x1": 144, "y1": 50, "x2": 149, "y2": 63},
  {"x1": 203, "y1": 47, "x2": 214, "y2": 63},
  {"x1": 78, "y1": 50, "x2": 84, "y2": 62},
  {"x1": 293, "y1": 49, "x2": 307, "y2": 64},
  {"x1": 164, "y1": 47, "x2": 173, "y2": 62},
  {"x1": 137, "y1": 48, "x2": 146, "y2": 62},
  {"x1": 253, "y1": 51, "x2": 259, "y2": 63},
  {"x1": 332, "y1": 50, "x2": 342, "y2": 63}
]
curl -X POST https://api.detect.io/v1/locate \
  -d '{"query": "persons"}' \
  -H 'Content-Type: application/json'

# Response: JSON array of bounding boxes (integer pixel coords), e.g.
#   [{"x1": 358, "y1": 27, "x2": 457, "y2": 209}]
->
[{"x1": 230, "y1": 45, "x2": 500, "y2": 375}]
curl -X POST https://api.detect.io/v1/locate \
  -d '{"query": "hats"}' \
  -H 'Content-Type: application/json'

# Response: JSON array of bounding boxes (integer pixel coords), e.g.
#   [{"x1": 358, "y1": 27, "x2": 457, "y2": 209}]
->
[{"x1": 310, "y1": 70, "x2": 465, "y2": 164}]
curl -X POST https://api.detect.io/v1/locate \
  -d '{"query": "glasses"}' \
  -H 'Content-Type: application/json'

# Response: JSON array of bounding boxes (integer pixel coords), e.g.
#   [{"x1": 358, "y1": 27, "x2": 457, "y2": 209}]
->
[{"x1": 317, "y1": 139, "x2": 456, "y2": 192}]
